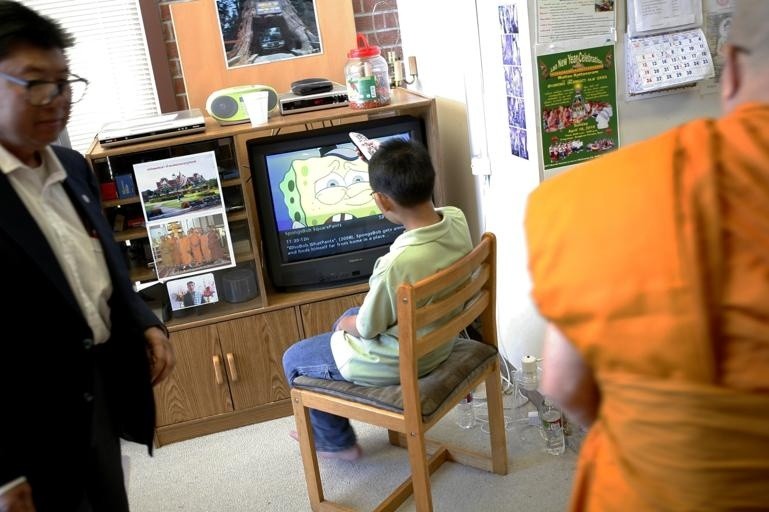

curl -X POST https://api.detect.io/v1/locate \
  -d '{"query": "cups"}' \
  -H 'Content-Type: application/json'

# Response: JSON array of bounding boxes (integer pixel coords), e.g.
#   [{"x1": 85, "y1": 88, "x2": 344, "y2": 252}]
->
[{"x1": 241, "y1": 91, "x2": 269, "y2": 128}]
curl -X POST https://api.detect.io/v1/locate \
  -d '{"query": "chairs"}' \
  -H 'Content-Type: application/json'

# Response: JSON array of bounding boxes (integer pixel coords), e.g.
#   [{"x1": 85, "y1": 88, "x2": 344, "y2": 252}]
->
[{"x1": 289, "y1": 233, "x2": 510, "y2": 511}]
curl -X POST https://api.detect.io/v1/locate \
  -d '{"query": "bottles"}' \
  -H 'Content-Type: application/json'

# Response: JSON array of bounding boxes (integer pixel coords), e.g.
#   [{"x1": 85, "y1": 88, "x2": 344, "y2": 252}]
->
[
  {"x1": 541, "y1": 397, "x2": 566, "y2": 456},
  {"x1": 455, "y1": 390, "x2": 477, "y2": 430}
]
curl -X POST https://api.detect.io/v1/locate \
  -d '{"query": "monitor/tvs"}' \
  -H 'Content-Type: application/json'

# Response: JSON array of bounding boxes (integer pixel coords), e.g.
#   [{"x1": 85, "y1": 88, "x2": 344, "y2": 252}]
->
[{"x1": 246, "y1": 114, "x2": 434, "y2": 293}]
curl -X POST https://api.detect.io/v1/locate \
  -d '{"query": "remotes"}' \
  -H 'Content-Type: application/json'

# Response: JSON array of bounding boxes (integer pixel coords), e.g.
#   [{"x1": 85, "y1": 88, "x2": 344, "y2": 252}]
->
[{"x1": 348, "y1": 131, "x2": 378, "y2": 161}]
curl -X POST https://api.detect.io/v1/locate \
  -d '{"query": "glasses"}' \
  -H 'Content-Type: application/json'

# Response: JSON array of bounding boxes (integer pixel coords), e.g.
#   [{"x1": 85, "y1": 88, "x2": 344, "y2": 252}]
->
[{"x1": 0, "y1": 71, "x2": 87, "y2": 106}]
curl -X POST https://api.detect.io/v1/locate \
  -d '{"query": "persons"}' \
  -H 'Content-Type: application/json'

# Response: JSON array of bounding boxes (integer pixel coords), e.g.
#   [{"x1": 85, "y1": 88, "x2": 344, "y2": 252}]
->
[
  {"x1": 542, "y1": 89, "x2": 615, "y2": 164},
  {"x1": 158, "y1": 223, "x2": 225, "y2": 274},
  {"x1": 0, "y1": 0, "x2": 177, "y2": 512},
  {"x1": 523, "y1": 1, "x2": 769, "y2": 511},
  {"x1": 499, "y1": 6, "x2": 528, "y2": 159},
  {"x1": 280, "y1": 136, "x2": 475, "y2": 462},
  {"x1": 182, "y1": 280, "x2": 207, "y2": 307}
]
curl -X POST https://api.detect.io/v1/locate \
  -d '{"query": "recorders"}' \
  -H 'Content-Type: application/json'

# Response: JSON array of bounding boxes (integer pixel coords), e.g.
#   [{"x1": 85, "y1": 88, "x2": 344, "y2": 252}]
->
[{"x1": 205, "y1": 85, "x2": 279, "y2": 126}]
